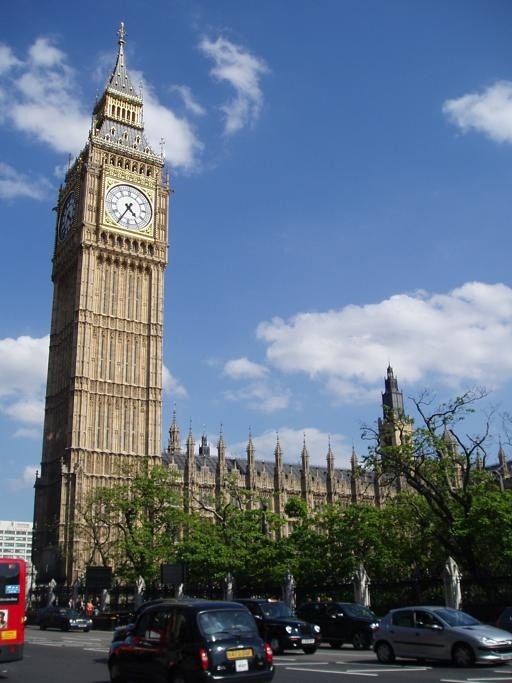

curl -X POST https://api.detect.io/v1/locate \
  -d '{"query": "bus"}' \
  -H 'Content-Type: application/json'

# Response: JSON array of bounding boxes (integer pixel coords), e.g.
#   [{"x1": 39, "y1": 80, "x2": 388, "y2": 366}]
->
[{"x1": 1, "y1": 554, "x2": 25, "y2": 664}]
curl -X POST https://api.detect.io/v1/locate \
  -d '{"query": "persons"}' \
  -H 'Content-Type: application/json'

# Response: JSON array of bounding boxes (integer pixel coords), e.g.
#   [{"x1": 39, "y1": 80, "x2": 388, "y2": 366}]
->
[
  {"x1": 306, "y1": 593, "x2": 313, "y2": 602},
  {"x1": 85, "y1": 598, "x2": 95, "y2": 617},
  {"x1": 315, "y1": 592, "x2": 321, "y2": 601},
  {"x1": 95, "y1": 595, "x2": 101, "y2": 607},
  {"x1": 266, "y1": 594, "x2": 280, "y2": 602},
  {"x1": 77, "y1": 597, "x2": 84, "y2": 615}
]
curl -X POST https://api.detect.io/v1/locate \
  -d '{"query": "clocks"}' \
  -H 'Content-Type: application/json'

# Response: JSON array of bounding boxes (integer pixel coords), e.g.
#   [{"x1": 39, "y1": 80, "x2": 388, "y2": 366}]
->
[
  {"x1": 58, "y1": 190, "x2": 76, "y2": 241},
  {"x1": 103, "y1": 182, "x2": 154, "y2": 231}
]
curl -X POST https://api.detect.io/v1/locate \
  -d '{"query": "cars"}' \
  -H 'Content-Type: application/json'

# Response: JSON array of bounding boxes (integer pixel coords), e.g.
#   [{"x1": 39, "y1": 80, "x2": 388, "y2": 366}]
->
[
  {"x1": 367, "y1": 605, "x2": 511, "y2": 668},
  {"x1": 106, "y1": 591, "x2": 383, "y2": 682},
  {"x1": 38, "y1": 607, "x2": 94, "y2": 633}
]
